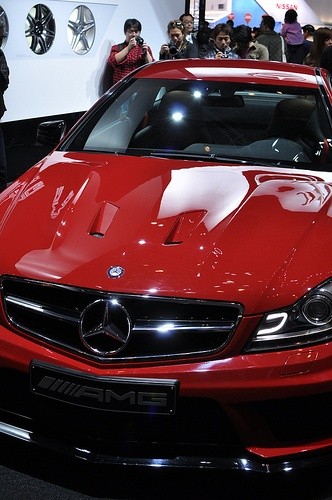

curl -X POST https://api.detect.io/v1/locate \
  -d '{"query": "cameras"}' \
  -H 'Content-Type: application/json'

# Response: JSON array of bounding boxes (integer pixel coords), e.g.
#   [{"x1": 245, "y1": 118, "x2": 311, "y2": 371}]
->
[
  {"x1": 135, "y1": 37, "x2": 144, "y2": 44},
  {"x1": 166, "y1": 43, "x2": 179, "y2": 54}
]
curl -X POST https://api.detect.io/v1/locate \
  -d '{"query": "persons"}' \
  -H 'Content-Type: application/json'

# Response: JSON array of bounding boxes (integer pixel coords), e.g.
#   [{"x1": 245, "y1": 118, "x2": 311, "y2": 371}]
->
[
  {"x1": 108, "y1": 18, "x2": 156, "y2": 86},
  {"x1": 159, "y1": 9, "x2": 332, "y2": 74},
  {"x1": 0, "y1": 23, "x2": 10, "y2": 193}
]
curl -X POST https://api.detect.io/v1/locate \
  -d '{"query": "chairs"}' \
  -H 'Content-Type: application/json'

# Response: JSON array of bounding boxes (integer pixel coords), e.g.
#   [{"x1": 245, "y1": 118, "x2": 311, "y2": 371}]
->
[
  {"x1": 266, "y1": 97, "x2": 330, "y2": 167},
  {"x1": 154, "y1": 90, "x2": 209, "y2": 154}
]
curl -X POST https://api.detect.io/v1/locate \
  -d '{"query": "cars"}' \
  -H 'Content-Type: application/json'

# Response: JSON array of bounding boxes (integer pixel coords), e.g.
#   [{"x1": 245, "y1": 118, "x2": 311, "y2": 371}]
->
[{"x1": 0, "y1": 57, "x2": 332, "y2": 500}]
